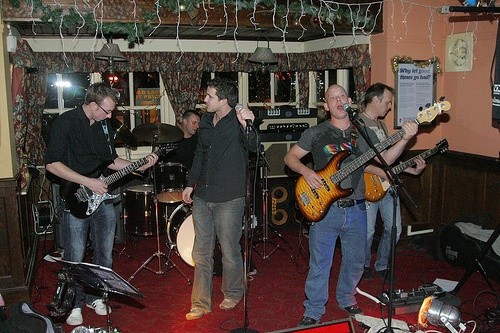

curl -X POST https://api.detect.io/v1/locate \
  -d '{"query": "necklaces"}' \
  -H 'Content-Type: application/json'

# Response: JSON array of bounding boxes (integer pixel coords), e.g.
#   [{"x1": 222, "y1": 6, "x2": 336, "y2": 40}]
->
[
  {"x1": 364, "y1": 110, "x2": 372, "y2": 118},
  {"x1": 329, "y1": 118, "x2": 336, "y2": 127}
]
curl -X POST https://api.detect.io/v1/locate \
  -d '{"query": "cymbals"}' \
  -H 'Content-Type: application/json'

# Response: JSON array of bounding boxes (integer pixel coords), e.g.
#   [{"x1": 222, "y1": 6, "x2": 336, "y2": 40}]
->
[
  {"x1": 108, "y1": 116, "x2": 139, "y2": 151},
  {"x1": 131, "y1": 122, "x2": 185, "y2": 149}
]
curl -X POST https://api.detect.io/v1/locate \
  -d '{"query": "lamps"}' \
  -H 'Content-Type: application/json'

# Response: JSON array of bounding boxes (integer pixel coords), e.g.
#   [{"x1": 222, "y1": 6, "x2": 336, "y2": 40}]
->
[
  {"x1": 246, "y1": 35, "x2": 279, "y2": 78},
  {"x1": 95, "y1": 41, "x2": 128, "y2": 74},
  {"x1": 418, "y1": 296, "x2": 477, "y2": 333}
]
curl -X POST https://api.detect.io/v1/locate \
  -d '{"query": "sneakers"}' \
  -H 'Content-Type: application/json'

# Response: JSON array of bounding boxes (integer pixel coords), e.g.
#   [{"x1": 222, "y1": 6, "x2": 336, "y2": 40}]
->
[
  {"x1": 65, "y1": 308, "x2": 83, "y2": 326},
  {"x1": 86, "y1": 298, "x2": 111, "y2": 315}
]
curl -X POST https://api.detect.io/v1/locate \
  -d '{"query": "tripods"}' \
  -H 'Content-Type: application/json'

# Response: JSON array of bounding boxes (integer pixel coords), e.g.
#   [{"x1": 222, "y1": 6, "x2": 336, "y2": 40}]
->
[
  {"x1": 126, "y1": 132, "x2": 189, "y2": 284},
  {"x1": 247, "y1": 151, "x2": 297, "y2": 260}
]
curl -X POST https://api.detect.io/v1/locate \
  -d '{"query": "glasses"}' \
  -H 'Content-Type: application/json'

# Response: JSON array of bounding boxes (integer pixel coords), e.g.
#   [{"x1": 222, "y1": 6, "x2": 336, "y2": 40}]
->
[{"x1": 92, "y1": 100, "x2": 112, "y2": 116}]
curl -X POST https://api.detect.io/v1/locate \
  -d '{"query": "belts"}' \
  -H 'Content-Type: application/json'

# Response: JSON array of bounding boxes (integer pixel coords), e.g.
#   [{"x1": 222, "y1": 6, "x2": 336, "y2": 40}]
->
[{"x1": 335, "y1": 198, "x2": 366, "y2": 208}]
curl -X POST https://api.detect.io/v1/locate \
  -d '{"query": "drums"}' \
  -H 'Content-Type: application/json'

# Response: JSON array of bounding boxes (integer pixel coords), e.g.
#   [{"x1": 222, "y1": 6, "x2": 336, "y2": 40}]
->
[
  {"x1": 121, "y1": 184, "x2": 163, "y2": 241},
  {"x1": 166, "y1": 203, "x2": 197, "y2": 269},
  {"x1": 156, "y1": 162, "x2": 187, "y2": 205}
]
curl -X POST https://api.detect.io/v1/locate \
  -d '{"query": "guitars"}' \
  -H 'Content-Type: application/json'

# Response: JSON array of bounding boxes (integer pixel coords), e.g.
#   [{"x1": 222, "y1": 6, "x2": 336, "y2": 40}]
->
[
  {"x1": 61, "y1": 141, "x2": 175, "y2": 219},
  {"x1": 295, "y1": 95, "x2": 452, "y2": 222},
  {"x1": 364, "y1": 137, "x2": 450, "y2": 203}
]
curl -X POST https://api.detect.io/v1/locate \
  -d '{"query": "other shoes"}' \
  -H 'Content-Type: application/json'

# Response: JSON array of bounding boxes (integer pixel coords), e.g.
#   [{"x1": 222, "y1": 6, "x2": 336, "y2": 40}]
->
[
  {"x1": 339, "y1": 304, "x2": 362, "y2": 315},
  {"x1": 297, "y1": 315, "x2": 317, "y2": 327},
  {"x1": 375, "y1": 268, "x2": 395, "y2": 282},
  {"x1": 361, "y1": 266, "x2": 372, "y2": 281},
  {"x1": 186, "y1": 307, "x2": 211, "y2": 320},
  {"x1": 218, "y1": 297, "x2": 241, "y2": 309}
]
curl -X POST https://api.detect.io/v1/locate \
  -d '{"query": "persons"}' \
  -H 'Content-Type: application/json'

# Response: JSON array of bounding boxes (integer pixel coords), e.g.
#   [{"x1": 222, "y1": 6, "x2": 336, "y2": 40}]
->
[
  {"x1": 182, "y1": 76, "x2": 261, "y2": 319},
  {"x1": 284, "y1": 84, "x2": 418, "y2": 325},
  {"x1": 168, "y1": 109, "x2": 201, "y2": 200},
  {"x1": 44, "y1": 82, "x2": 158, "y2": 325},
  {"x1": 354, "y1": 83, "x2": 426, "y2": 282}
]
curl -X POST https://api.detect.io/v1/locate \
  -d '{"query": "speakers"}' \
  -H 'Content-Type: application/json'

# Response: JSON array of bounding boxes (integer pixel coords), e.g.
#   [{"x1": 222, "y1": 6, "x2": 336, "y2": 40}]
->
[{"x1": 253, "y1": 173, "x2": 293, "y2": 230}]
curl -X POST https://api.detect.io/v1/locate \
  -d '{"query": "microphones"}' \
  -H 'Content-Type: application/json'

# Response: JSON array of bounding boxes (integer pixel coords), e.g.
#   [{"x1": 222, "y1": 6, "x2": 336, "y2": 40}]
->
[
  {"x1": 234, "y1": 103, "x2": 251, "y2": 126},
  {"x1": 342, "y1": 103, "x2": 363, "y2": 123}
]
